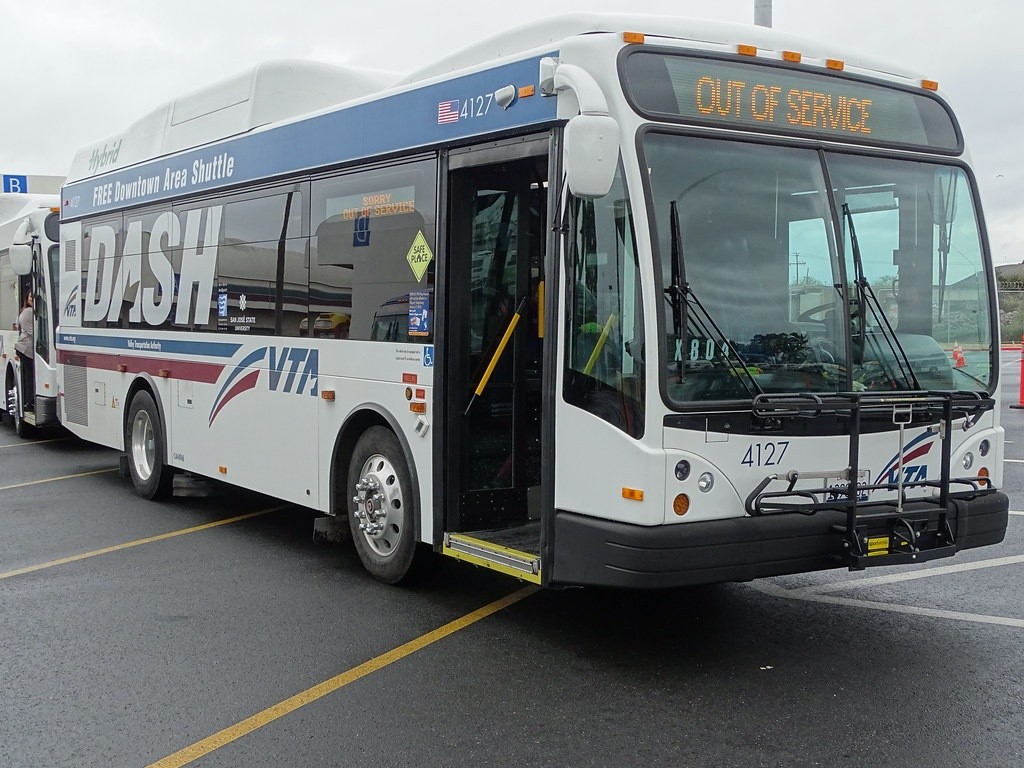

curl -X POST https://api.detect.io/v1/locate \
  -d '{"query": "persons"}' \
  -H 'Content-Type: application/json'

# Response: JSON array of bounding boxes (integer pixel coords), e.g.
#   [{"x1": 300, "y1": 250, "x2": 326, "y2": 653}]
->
[{"x1": 14, "y1": 289, "x2": 35, "y2": 411}]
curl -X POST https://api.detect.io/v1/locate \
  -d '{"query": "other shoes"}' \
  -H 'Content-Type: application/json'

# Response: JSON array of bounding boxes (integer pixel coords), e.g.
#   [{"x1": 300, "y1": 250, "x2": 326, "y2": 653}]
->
[{"x1": 24, "y1": 403, "x2": 34, "y2": 412}]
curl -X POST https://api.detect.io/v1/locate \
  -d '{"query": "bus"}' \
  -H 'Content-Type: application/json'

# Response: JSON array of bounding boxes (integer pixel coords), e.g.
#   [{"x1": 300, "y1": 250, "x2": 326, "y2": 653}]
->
[
  {"x1": 0, "y1": 171, "x2": 69, "y2": 440},
  {"x1": 47, "y1": 12, "x2": 1010, "y2": 589}
]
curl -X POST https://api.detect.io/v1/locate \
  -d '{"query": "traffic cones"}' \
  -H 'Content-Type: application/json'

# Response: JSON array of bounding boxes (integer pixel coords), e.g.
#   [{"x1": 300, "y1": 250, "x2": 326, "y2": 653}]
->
[{"x1": 951, "y1": 336, "x2": 967, "y2": 369}]
